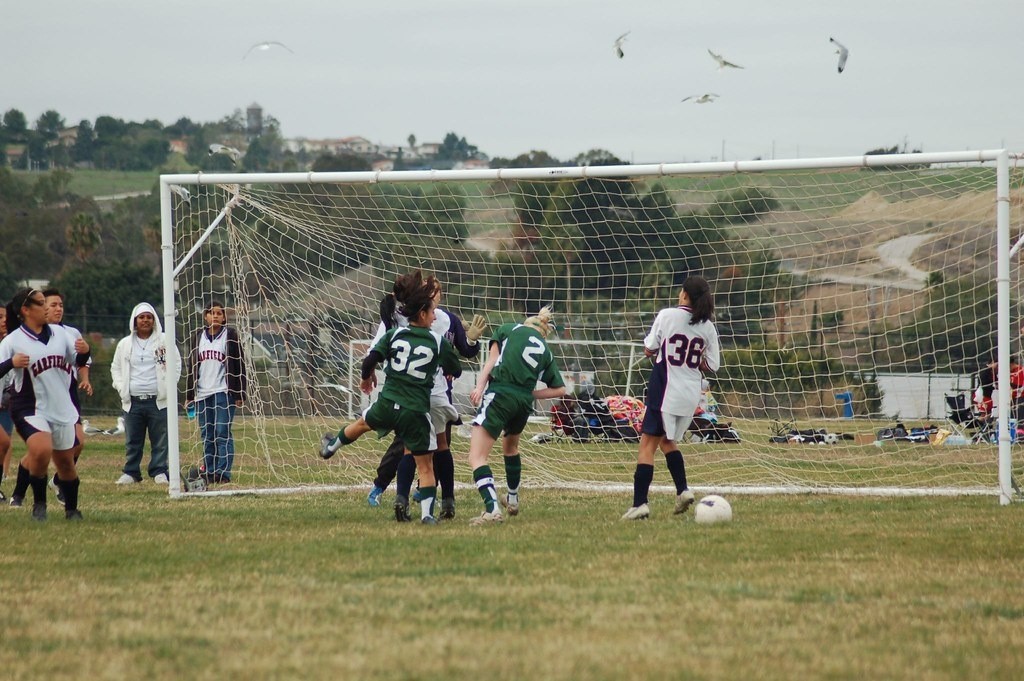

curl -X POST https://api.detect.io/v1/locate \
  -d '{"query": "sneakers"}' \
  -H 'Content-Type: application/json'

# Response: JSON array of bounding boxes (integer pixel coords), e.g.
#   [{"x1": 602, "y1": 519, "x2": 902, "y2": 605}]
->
[
  {"x1": 115, "y1": 474, "x2": 138, "y2": 483},
  {"x1": 439, "y1": 511, "x2": 455, "y2": 520},
  {"x1": 48, "y1": 473, "x2": 65, "y2": 505},
  {"x1": 500, "y1": 494, "x2": 518, "y2": 516},
  {"x1": 622, "y1": 503, "x2": 649, "y2": 519},
  {"x1": 319, "y1": 432, "x2": 335, "y2": 460},
  {"x1": 422, "y1": 516, "x2": 436, "y2": 524},
  {"x1": 65, "y1": 509, "x2": 82, "y2": 520},
  {"x1": 32, "y1": 503, "x2": 46, "y2": 522},
  {"x1": 672, "y1": 489, "x2": 694, "y2": 515},
  {"x1": 413, "y1": 491, "x2": 421, "y2": 505},
  {"x1": 393, "y1": 494, "x2": 411, "y2": 523},
  {"x1": 154, "y1": 473, "x2": 168, "y2": 484},
  {"x1": 9, "y1": 496, "x2": 21, "y2": 507},
  {"x1": 469, "y1": 510, "x2": 505, "y2": 527},
  {"x1": 366, "y1": 486, "x2": 382, "y2": 506}
]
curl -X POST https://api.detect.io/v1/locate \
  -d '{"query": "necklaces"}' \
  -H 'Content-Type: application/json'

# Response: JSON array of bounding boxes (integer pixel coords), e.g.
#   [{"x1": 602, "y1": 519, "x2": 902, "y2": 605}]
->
[{"x1": 136, "y1": 336, "x2": 149, "y2": 350}]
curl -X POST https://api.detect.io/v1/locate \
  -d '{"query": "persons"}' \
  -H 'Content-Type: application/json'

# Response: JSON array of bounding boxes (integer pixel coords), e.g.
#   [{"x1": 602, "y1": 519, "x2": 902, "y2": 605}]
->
[
  {"x1": 0, "y1": 288, "x2": 91, "y2": 519},
  {"x1": 695, "y1": 380, "x2": 733, "y2": 427},
  {"x1": 109, "y1": 301, "x2": 182, "y2": 483},
  {"x1": 577, "y1": 381, "x2": 609, "y2": 417},
  {"x1": 10, "y1": 288, "x2": 94, "y2": 509},
  {"x1": 620, "y1": 275, "x2": 720, "y2": 519},
  {"x1": 185, "y1": 301, "x2": 247, "y2": 483},
  {"x1": 469, "y1": 305, "x2": 566, "y2": 526},
  {"x1": 0, "y1": 302, "x2": 14, "y2": 502},
  {"x1": 972, "y1": 355, "x2": 1024, "y2": 443},
  {"x1": 318, "y1": 269, "x2": 487, "y2": 523},
  {"x1": 554, "y1": 394, "x2": 589, "y2": 442}
]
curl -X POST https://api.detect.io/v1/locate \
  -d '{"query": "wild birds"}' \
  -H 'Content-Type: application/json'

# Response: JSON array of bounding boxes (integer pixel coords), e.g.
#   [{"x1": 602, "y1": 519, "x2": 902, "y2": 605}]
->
[
  {"x1": 241, "y1": 41, "x2": 292, "y2": 60},
  {"x1": 208, "y1": 143, "x2": 240, "y2": 169},
  {"x1": 706, "y1": 48, "x2": 746, "y2": 72},
  {"x1": 81, "y1": 417, "x2": 126, "y2": 437},
  {"x1": 612, "y1": 30, "x2": 632, "y2": 59},
  {"x1": 682, "y1": 92, "x2": 721, "y2": 104},
  {"x1": 829, "y1": 36, "x2": 849, "y2": 74}
]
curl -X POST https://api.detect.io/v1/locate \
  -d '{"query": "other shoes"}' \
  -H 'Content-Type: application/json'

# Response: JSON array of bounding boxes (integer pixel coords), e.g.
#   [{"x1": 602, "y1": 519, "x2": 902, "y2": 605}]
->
[
  {"x1": 201, "y1": 470, "x2": 231, "y2": 484},
  {"x1": 0, "y1": 491, "x2": 7, "y2": 503}
]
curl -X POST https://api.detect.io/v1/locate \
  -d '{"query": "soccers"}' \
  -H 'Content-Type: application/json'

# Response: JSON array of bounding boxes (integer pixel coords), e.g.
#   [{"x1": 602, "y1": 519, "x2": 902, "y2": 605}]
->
[
  {"x1": 692, "y1": 493, "x2": 733, "y2": 525},
  {"x1": 823, "y1": 433, "x2": 840, "y2": 446}
]
